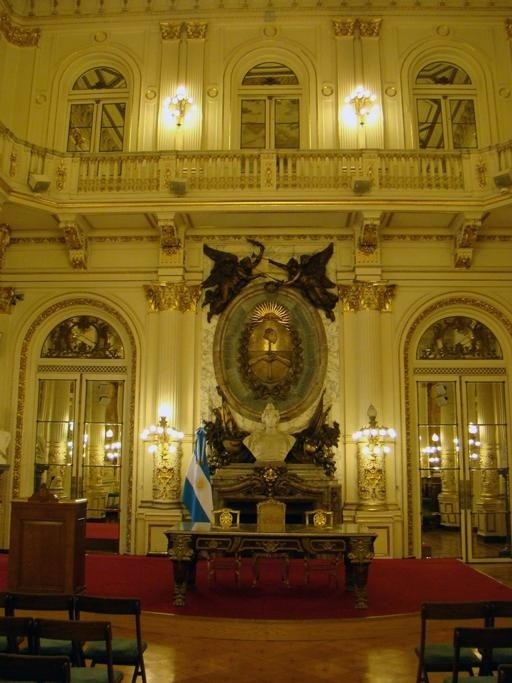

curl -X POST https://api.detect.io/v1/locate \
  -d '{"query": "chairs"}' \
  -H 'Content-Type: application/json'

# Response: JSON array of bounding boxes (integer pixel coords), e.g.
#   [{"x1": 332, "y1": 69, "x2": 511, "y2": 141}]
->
[
  {"x1": 414, "y1": 600, "x2": 512, "y2": 683},
  {"x1": 209, "y1": 508, "x2": 241, "y2": 594},
  {"x1": 0, "y1": 591, "x2": 148, "y2": 683},
  {"x1": 304, "y1": 509, "x2": 339, "y2": 590},
  {"x1": 251, "y1": 495, "x2": 290, "y2": 590}
]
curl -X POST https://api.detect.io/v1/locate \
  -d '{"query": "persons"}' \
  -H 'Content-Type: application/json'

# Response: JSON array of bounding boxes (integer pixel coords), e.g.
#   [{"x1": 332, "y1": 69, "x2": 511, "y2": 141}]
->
[
  {"x1": 281, "y1": 243, "x2": 338, "y2": 323},
  {"x1": 242, "y1": 402, "x2": 296, "y2": 462},
  {"x1": 198, "y1": 244, "x2": 268, "y2": 323}
]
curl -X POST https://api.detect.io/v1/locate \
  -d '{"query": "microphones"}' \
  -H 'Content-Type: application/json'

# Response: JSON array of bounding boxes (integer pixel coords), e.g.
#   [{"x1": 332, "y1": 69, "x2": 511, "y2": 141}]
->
[{"x1": 47, "y1": 475, "x2": 55, "y2": 493}]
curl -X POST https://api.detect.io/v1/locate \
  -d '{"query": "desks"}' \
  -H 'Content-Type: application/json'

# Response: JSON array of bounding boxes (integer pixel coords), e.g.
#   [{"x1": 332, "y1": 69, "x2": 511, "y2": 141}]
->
[{"x1": 163, "y1": 522, "x2": 378, "y2": 609}]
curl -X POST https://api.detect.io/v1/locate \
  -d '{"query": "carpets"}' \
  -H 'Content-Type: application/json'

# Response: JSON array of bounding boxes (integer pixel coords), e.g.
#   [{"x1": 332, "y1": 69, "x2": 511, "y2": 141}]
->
[{"x1": 0, "y1": 552, "x2": 511, "y2": 620}]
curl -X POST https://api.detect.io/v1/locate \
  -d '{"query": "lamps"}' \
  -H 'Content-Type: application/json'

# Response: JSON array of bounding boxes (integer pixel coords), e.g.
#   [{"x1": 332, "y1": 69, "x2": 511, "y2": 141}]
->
[
  {"x1": 164, "y1": 84, "x2": 194, "y2": 125},
  {"x1": 352, "y1": 403, "x2": 396, "y2": 460},
  {"x1": 168, "y1": 178, "x2": 188, "y2": 195},
  {"x1": 140, "y1": 403, "x2": 184, "y2": 460},
  {"x1": 344, "y1": 84, "x2": 376, "y2": 125},
  {"x1": 351, "y1": 175, "x2": 370, "y2": 193},
  {"x1": 28, "y1": 172, "x2": 51, "y2": 193},
  {"x1": 492, "y1": 168, "x2": 512, "y2": 189}
]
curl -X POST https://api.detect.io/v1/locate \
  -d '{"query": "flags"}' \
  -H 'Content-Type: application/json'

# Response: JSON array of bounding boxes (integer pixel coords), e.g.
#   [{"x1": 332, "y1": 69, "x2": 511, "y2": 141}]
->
[{"x1": 182, "y1": 429, "x2": 215, "y2": 532}]
